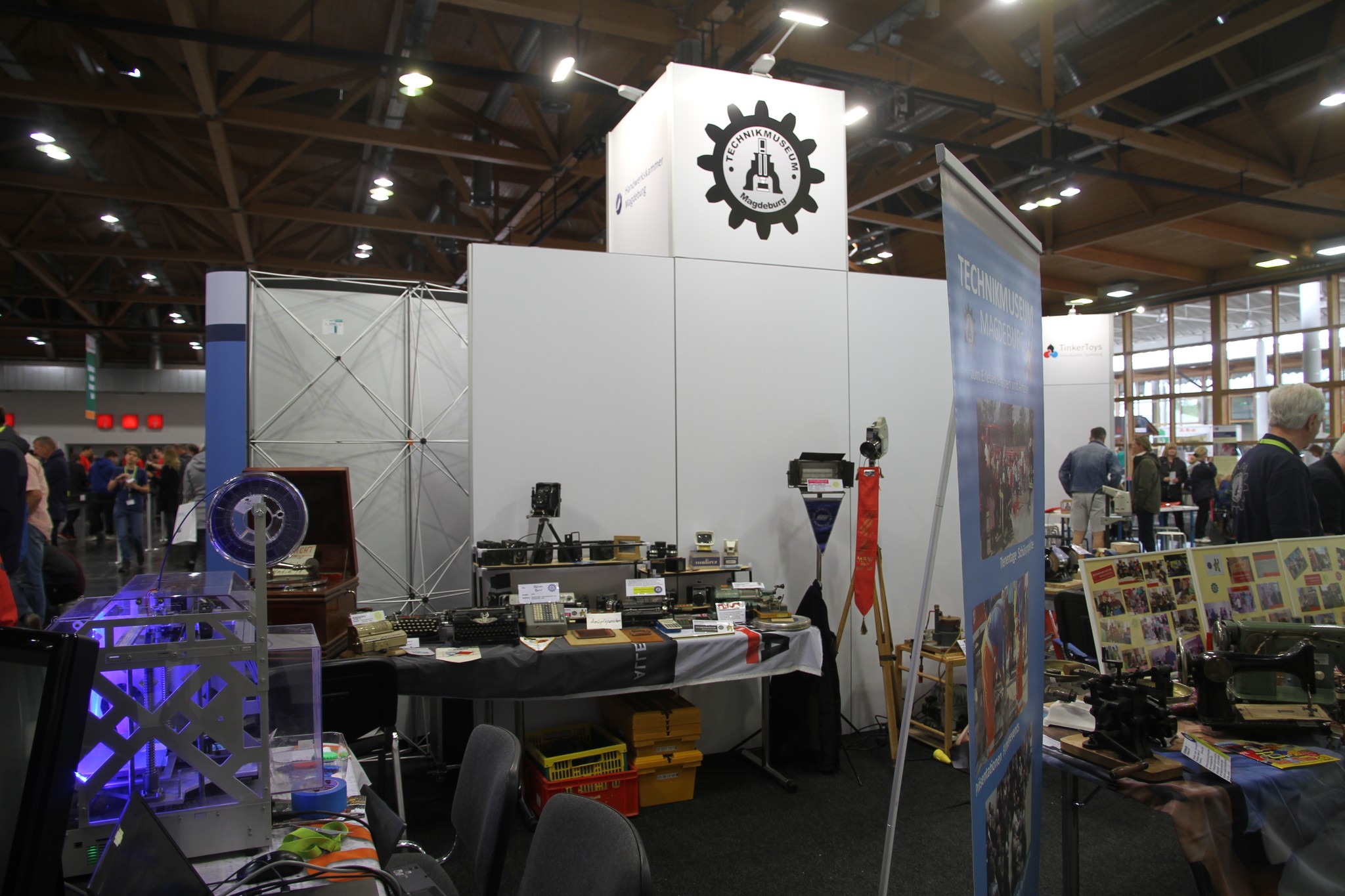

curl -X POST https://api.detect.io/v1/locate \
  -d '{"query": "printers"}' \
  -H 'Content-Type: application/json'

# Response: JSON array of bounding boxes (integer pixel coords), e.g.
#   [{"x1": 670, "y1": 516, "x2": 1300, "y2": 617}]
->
[{"x1": 346, "y1": 605, "x2": 520, "y2": 657}]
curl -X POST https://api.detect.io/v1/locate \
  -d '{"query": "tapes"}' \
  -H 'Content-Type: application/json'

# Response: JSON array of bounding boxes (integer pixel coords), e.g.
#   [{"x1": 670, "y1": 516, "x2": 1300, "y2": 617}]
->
[{"x1": 291, "y1": 775, "x2": 347, "y2": 820}]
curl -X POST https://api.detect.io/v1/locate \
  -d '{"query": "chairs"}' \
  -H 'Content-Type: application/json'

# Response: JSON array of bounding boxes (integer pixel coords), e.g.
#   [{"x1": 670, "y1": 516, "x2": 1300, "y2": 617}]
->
[
  {"x1": 360, "y1": 724, "x2": 653, "y2": 896},
  {"x1": 270, "y1": 654, "x2": 399, "y2": 748}
]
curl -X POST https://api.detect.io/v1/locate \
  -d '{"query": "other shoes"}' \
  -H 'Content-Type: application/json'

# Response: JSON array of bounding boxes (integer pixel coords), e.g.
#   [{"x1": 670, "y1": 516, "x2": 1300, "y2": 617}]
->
[
  {"x1": 1194, "y1": 536, "x2": 1211, "y2": 543},
  {"x1": 25, "y1": 613, "x2": 42, "y2": 630},
  {"x1": 57, "y1": 532, "x2": 78, "y2": 541}
]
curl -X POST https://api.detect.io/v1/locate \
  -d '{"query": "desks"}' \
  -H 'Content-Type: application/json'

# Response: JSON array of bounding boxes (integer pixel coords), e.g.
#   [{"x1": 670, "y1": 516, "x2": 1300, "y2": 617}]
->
[
  {"x1": 1042, "y1": 695, "x2": 1345, "y2": 896},
  {"x1": 894, "y1": 643, "x2": 966, "y2": 757},
  {"x1": 184, "y1": 743, "x2": 388, "y2": 896},
  {"x1": 333, "y1": 619, "x2": 825, "y2": 794},
  {"x1": 1045, "y1": 504, "x2": 1200, "y2": 553}
]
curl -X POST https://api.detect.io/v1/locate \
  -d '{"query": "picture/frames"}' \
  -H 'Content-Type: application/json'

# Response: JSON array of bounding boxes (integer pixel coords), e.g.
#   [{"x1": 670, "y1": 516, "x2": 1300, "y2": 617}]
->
[{"x1": 1060, "y1": 499, "x2": 1073, "y2": 514}]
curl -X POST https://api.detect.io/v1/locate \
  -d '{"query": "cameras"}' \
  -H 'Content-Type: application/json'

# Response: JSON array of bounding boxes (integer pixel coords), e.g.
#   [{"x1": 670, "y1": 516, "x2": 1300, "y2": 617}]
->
[
  {"x1": 859, "y1": 417, "x2": 889, "y2": 460},
  {"x1": 687, "y1": 579, "x2": 716, "y2": 606},
  {"x1": 596, "y1": 594, "x2": 624, "y2": 612},
  {"x1": 574, "y1": 594, "x2": 590, "y2": 611},
  {"x1": 477, "y1": 539, "x2": 528, "y2": 566},
  {"x1": 589, "y1": 541, "x2": 614, "y2": 561},
  {"x1": 530, "y1": 482, "x2": 561, "y2": 517},
  {"x1": 488, "y1": 591, "x2": 513, "y2": 607}
]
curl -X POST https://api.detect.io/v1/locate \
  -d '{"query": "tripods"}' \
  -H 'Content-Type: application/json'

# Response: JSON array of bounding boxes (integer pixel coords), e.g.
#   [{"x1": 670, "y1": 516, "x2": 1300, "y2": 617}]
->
[{"x1": 529, "y1": 518, "x2": 575, "y2": 566}]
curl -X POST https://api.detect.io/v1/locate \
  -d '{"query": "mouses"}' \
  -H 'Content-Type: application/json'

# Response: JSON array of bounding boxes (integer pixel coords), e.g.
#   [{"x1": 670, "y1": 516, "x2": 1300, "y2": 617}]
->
[{"x1": 236, "y1": 850, "x2": 306, "y2": 885}]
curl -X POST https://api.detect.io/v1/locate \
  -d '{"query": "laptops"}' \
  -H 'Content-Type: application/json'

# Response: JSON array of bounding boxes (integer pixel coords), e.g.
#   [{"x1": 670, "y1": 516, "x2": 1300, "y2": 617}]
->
[{"x1": 86, "y1": 790, "x2": 379, "y2": 896}]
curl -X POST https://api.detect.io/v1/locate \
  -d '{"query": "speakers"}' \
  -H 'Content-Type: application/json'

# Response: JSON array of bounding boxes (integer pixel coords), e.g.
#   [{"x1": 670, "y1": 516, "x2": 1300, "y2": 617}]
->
[
  {"x1": 533, "y1": 541, "x2": 553, "y2": 564},
  {"x1": 557, "y1": 532, "x2": 583, "y2": 563}
]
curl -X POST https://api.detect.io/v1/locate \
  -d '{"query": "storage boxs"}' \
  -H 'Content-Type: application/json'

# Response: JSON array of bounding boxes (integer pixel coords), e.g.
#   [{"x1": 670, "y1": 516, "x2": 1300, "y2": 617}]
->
[
  {"x1": 512, "y1": 686, "x2": 704, "y2": 818},
  {"x1": 266, "y1": 732, "x2": 352, "y2": 801}
]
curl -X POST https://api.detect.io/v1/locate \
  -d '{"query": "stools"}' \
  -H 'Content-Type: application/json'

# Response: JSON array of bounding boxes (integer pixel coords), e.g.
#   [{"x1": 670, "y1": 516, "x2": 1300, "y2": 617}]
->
[{"x1": 1110, "y1": 527, "x2": 1187, "y2": 552}]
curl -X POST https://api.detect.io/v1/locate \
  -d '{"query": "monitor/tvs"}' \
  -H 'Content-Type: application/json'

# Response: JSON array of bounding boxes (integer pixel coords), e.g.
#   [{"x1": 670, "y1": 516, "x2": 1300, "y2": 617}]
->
[{"x1": 0, "y1": 628, "x2": 100, "y2": 896}]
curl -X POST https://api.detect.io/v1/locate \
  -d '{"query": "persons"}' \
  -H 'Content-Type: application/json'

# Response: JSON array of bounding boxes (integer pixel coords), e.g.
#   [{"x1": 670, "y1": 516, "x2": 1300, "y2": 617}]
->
[
  {"x1": 1306, "y1": 431, "x2": 1345, "y2": 537},
  {"x1": 1115, "y1": 447, "x2": 1125, "y2": 474},
  {"x1": 1213, "y1": 475, "x2": 1236, "y2": 540},
  {"x1": 1228, "y1": 383, "x2": 1326, "y2": 544},
  {"x1": 976, "y1": 397, "x2": 1032, "y2": 896},
  {"x1": 1186, "y1": 446, "x2": 1217, "y2": 543},
  {"x1": 1063, "y1": 503, "x2": 1071, "y2": 511},
  {"x1": 1131, "y1": 436, "x2": 1162, "y2": 552},
  {"x1": 0, "y1": 404, "x2": 206, "y2": 631},
  {"x1": 1058, "y1": 427, "x2": 1123, "y2": 549},
  {"x1": 1086, "y1": 544, "x2": 1345, "y2": 674},
  {"x1": 1158, "y1": 442, "x2": 1188, "y2": 540}
]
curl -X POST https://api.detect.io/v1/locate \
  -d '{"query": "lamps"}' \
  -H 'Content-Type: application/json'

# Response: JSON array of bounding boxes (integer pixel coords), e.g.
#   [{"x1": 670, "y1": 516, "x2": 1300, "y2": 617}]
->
[
  {"x1": 552, "y1": 57, "x2": 647, "y2": 103},
  {"x1": 1052, "y1": 52, "x2": 1099, "y2": 120},
  {"x1": 354, "y1": 25, "x2": 548, "y2": 259},
  {"x1": 1249, "y1": 234, "x2": 1345, "y2": 269},
  {"x1": 747, "y1": 7, "x2": 830, "y2": 79},
  {"x1": 723, "y1": 452, "x2": 866, "y2": 791},
  {"x1": 848, "y1": 170, "x2": 1138, "y2": 305}
]
